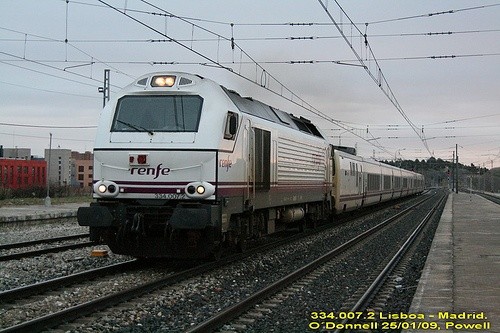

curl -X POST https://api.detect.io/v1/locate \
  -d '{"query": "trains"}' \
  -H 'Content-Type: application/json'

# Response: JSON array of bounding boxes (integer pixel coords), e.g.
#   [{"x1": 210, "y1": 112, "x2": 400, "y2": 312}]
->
[{"x1": 77, "y1": 71, "x2": 425, "y2": 255}]
